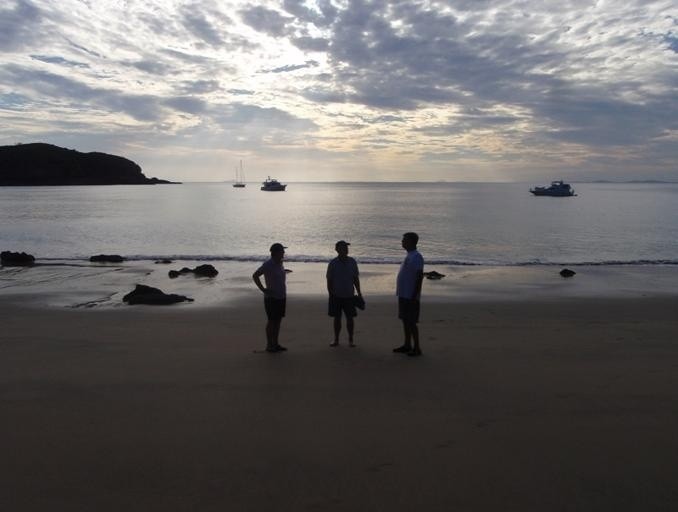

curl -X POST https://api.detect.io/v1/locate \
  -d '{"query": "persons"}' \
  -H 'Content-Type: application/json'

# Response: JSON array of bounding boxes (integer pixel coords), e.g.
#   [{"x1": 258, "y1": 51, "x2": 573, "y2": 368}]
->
[
  {"x1": 327, "y1": 240, "x2": 362, "y2": 347},
  {"x1": 393, "y1": 231, "x2": 424, "y2": 356},
  {"x1": 253, "y1": 244, "x2": 287, "y2": 352}
]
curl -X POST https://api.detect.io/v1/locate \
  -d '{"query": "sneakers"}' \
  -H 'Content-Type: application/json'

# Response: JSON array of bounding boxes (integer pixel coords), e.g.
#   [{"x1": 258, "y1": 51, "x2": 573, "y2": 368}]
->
[
  {"x1": 392, "y1": 347, "x2": 411, "y2": 353},
  {"x1": 267, "y1": 344, "x2": 288, "y2": 352},
  {"x1": 408, "y1": 350, "x2": 422, "y2": 356},
  {"x1": 330, "y1": 340, "x2": 339, "y2": 346}
]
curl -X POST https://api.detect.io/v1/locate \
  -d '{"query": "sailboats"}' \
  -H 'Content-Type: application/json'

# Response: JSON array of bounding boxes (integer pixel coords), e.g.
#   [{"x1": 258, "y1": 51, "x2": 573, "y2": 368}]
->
[{"x1": 231, "y1": 160, "x2": 246, "y2": 187}]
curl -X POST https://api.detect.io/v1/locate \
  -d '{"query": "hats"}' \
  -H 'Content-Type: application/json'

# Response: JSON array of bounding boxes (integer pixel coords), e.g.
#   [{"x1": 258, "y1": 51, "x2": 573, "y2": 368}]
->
[
  {"x1": 335, "y1": 241, "x2": 351, "y2": 245},
  {"x1": 269, "y1": 242, "x2": 288, "y2": 250}
]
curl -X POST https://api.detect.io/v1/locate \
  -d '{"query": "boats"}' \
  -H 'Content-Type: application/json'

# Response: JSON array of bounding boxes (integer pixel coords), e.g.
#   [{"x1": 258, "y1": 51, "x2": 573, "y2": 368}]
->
[
  {"x1": 529, "y1": 181, "x2": 575, "y2": 197},
  {"x1": 260, "y1": 176, "x2": 286, "y2": 191}
]
